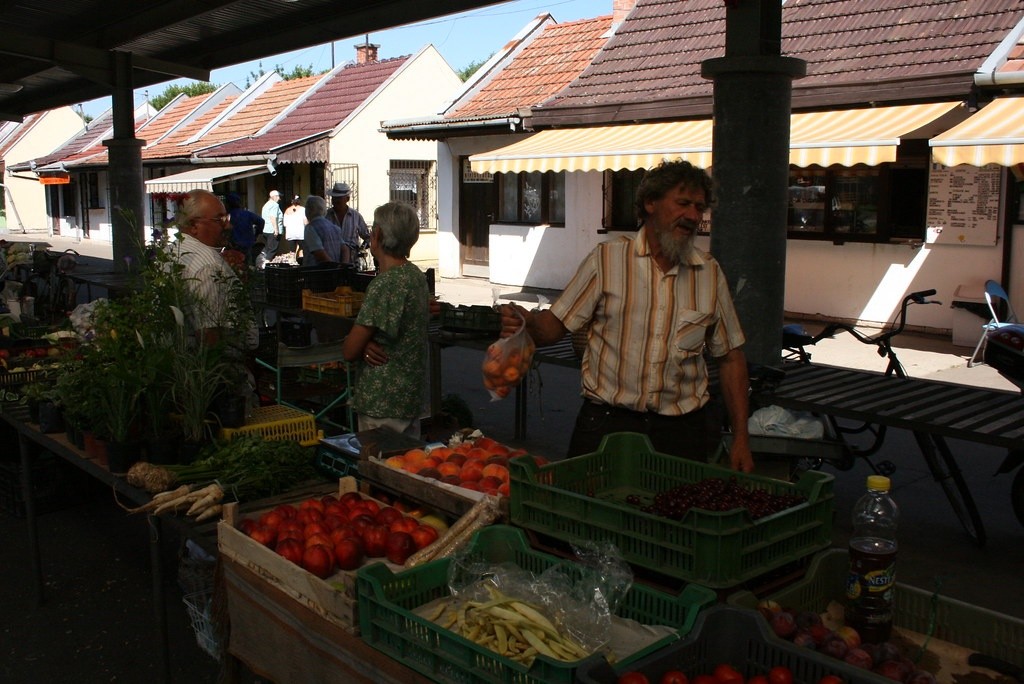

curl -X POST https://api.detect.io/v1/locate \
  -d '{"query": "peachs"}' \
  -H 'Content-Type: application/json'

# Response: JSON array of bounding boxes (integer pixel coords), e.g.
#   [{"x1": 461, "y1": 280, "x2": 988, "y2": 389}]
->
[
  {"x1": 385, "y1": 438, "x2": 550, "y2": 496},
  {"x1": 238, "y1": 492, "x2": 439, "y2": 572}
]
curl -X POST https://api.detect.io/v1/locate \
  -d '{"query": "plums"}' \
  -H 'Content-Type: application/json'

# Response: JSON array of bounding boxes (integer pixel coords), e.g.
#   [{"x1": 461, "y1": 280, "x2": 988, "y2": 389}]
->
[{"x1": 758, "y1": 600, "x2": 937, "y2": 684}]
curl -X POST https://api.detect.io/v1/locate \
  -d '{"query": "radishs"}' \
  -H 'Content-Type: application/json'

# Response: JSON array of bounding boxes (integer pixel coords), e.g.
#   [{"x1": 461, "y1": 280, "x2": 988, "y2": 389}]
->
[{"x1": 127, "y1": 484, "x2": 223, "y2": 520}]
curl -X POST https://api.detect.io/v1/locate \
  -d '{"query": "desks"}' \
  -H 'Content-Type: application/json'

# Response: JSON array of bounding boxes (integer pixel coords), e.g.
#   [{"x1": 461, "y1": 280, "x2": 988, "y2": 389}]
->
[
  {"x1": 57, "y1": 267, "x2": 134, "y2": 302},
  {"x1": 0, "y1": 404, "x2": 338, "y2": 684},
  {"x1": 522, "y1": 334, "x2": 1024, "y2": 455},
  {"x1": 426, "y1": 318, "x2": 521, "y2": 444}
]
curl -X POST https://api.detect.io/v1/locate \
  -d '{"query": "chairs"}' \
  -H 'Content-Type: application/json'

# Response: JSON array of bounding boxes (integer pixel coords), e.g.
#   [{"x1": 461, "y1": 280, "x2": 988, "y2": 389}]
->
[
  {"x1": 966, "y1": 279, "x2": 1024, "y2": 367},
  {"x1": 252, "y1": 338, "x2": 354, "y2": 434}
]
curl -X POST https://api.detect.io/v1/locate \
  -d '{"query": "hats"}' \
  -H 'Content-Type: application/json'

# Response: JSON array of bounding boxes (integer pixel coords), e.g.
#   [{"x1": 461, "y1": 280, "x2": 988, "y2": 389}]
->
[{"x1": 327, "y1": 183, "x2": 349, "y2": 197}]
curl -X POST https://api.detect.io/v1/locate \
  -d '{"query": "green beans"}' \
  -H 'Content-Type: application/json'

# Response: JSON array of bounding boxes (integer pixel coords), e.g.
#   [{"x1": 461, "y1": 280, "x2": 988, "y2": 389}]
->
[{"x1": 406, "y1": 585, "x2": 619, "y2": 683}]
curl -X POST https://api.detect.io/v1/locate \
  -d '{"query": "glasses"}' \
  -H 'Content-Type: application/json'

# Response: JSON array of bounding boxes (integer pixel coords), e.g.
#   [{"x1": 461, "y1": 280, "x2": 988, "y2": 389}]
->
[{"x1": 195, "y1": 213, "x2": 231, "y2": 225}]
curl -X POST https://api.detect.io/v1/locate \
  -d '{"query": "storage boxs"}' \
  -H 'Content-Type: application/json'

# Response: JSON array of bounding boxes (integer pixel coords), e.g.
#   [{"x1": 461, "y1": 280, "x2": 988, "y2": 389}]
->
[{"x1": 0, "y1": 264, "x2": 1024, "y2": 684}]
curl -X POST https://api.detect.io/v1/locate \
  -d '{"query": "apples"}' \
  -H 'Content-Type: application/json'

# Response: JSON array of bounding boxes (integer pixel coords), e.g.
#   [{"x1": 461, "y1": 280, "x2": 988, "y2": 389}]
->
[
  {"x1": 484, "y1": 343, "x2": 534, "y2": 398},
  {"x1": 0, "y1": 343, "x2": 77, "y2": 367}
]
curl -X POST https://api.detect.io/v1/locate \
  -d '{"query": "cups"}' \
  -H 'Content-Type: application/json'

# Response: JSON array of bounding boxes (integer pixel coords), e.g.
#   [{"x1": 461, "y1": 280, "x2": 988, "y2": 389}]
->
[{"x1": 7, "y1": 296, "x2": 35, "y2": 321}]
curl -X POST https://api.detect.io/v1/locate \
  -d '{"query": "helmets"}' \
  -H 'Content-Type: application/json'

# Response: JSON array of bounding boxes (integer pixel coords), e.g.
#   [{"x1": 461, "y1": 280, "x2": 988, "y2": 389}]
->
[{"x1": 226, "y1": 191, "x2": 239, "y2": 203}]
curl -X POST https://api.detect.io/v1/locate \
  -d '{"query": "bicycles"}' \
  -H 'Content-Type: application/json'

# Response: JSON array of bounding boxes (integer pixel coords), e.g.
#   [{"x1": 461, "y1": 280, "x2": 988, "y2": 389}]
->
[
  {"x1": 767, "y1": 288, "x2": 987, "y2": 546},
  {"x1": 19, "y1": 247, "x2": 81, "y2": 323},
  {"x1": 341, "y1": 235, "x2": 370, "y2": 271}
]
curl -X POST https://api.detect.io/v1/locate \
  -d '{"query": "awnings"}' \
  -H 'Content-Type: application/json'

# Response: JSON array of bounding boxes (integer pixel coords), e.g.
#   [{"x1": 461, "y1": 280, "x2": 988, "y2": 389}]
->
[
  {"x1": 144, "y1": 163, "x2": 270, "y2": 195},
  {"x1": 927, "y1": 94, "x2": 1024, "y2": 169},
  {"x1": 469, "y1": 99, "x2": 964, "y2": 177}
]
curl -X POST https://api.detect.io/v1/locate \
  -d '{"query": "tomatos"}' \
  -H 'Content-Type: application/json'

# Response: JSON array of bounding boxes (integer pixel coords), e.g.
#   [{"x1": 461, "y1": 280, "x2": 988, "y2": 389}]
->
[{"x1": 621, "y1": 663, "x2": 844, "y2": 684}]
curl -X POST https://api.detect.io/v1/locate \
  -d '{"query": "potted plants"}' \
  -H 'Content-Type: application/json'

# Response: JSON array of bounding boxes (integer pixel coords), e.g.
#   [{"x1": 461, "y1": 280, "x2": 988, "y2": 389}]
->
[{"x1": 19, "y1": 205, "x2": 240, "y2": 472}]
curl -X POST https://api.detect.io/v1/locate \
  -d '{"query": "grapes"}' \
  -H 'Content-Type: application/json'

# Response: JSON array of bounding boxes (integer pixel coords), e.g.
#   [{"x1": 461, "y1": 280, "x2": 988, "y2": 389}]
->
[{"x1": 625, "y1": 477, "x2": 806, "y2": 523}]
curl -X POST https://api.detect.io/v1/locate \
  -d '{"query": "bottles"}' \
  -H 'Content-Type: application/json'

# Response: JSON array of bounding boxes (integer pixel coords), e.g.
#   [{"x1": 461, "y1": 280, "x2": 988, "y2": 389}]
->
[{"x1": 842, "y1": 475, "x2": 899, "y2": 644}]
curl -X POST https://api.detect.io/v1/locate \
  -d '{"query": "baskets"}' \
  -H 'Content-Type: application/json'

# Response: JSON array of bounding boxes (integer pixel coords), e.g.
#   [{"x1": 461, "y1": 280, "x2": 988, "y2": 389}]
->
[
  {"x1": 225, "y1": 404, "x2": 324, "y2": 447},
  {"x1": 355, "y1": 433, "x2": 1024, "y2": 684}
]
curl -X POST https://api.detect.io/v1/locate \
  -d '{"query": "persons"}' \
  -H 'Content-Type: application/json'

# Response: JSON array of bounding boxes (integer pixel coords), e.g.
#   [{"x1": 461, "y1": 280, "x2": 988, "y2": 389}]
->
[
  {"x1": 342, "y1": 200, "x2": 432, "y2": 448},
  {"x1": 260, "y1": 189, "x2": 284, "y2": 261},
  {"x1": 302, "y1": 195, "x2": 351, "y2": 268},
  {"x1": 497, "y1": 160, "x2": 757, "y2": 475},
  {"x1": 226, "y1": 191, "x2": 265, "y2": 261},
  {"x1": 284, "y1": 194, "x2": 312, "y2": 258},
  {"x1": 169, "y1": 188, "x2": 261, "y2": 409},
  {"x1": 324, "y1": 183, "x2": 371, "y2": 272}
]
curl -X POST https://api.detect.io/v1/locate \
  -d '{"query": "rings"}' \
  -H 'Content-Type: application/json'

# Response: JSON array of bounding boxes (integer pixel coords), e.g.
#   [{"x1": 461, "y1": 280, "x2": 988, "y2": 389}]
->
[{"x1": 365, "y1": 354, "x2": 370, "y2": 358}]
